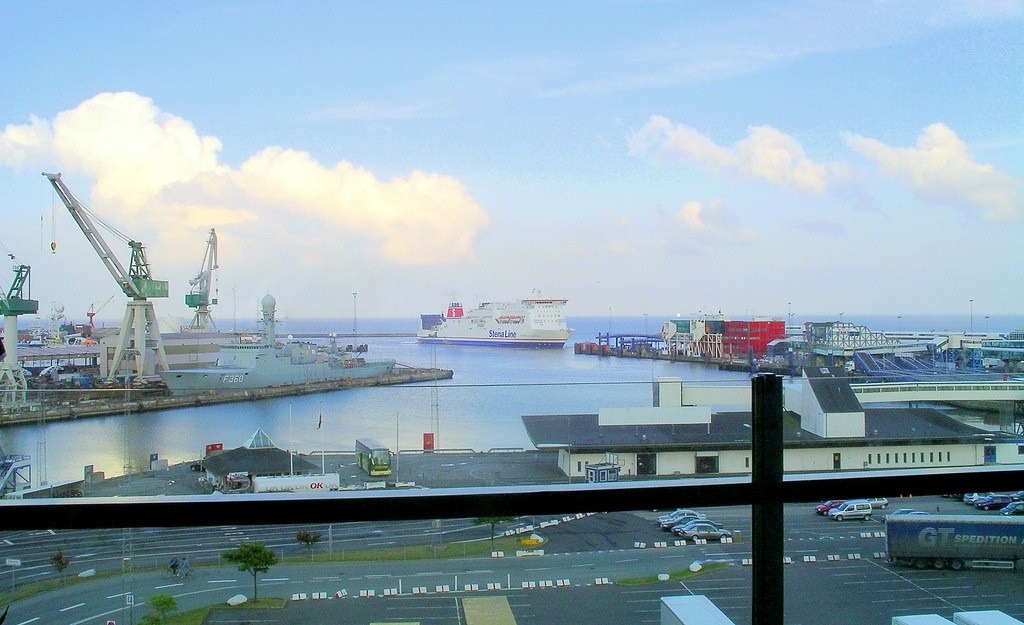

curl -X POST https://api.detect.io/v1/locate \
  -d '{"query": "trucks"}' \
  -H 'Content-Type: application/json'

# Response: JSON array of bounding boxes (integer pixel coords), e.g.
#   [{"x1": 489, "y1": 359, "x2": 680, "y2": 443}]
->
[{"x1": 845, "y1": 360, "x2": 862, "y2": 373}]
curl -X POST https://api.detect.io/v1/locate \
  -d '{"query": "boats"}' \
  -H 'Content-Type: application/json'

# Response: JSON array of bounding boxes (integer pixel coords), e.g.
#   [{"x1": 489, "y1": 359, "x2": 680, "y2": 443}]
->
[
  {"x1": 510, "y1": 315, "x2": 521, "y2": 323},
  {"x1": 157, "y1": 290, "x2": 397, "y2": 395},
  {"x1": 496, "y1": 316, "x2": 510, "y2": 323},
  {"x1": 342, "y1": 361, "x2": 358, "y2": 368}
]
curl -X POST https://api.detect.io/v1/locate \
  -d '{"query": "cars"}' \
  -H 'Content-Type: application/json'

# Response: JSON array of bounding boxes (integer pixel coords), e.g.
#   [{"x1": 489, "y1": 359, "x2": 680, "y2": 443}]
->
[
  {"x1": 657, "y1": 509, "x2": 706, "y2": 525},
  {"x1": 671, "y1": 519, "x2": 723, "y2": 536},
  {"x1": 908, "y1": 512, "x2": 929, "y2": 516},
  {"x1": 679, "y1": 524, "x2": 732, "y2": 542},
  {"x1": 661, "y1": 516, "x2": 701, "y2": 532},
  {"x1": 881, "y1": 509, "x2": 916, "y2": 522},
  {"x1": 1009, "y1": 491, "x2": 1024, "y2": 501},
  {"x1": 866, "y1": 498, "x2": 888, "y2": 509}
]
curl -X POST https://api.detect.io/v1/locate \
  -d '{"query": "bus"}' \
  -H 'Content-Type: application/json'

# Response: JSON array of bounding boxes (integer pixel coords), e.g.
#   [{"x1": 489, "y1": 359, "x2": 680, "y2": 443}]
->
[{"x1": 355, "y1": 438, "x2": 394, "y2": 476}]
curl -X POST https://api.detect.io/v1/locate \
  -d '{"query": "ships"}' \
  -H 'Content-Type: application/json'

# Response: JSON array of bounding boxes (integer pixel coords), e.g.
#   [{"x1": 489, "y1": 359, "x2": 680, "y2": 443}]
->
[{"x1": 417, "y1": 288, "x2": 575, "y2": 349}]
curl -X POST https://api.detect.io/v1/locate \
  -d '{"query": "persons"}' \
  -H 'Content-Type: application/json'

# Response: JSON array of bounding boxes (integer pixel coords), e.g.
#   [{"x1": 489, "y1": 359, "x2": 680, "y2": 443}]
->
[{"x1": 168, "y1": 555, "x2": 190, "y2": 578}]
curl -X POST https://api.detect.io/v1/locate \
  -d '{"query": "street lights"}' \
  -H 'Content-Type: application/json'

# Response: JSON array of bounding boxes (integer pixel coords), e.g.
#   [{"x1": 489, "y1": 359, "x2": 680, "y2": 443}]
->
[
  {"x1": 969, "y1": 299, "x2": 973, "y2": 333},
  {"x1": 897, "y1": 315, "x2": 903, "y2": 332},
  {"x1": 642, "y1": 313, "x2": 648, "y2": 334},
  {"x1": 787, "y1": 302, "x2": 794, "y2": 326},
  {"x1": 352, "y1": 292, "x2": 357, "y2": 332}
]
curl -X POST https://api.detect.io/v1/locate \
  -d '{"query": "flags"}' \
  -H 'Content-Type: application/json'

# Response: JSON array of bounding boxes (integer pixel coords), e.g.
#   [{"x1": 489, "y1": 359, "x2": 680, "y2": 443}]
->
[{"x1": 314, "y1": 410, "x2": 322, "y2": 430}]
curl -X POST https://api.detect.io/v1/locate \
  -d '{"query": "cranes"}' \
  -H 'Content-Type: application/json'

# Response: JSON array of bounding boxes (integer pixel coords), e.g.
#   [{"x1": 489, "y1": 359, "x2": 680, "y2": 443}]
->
[
  {"x1": 0, "y1": 241, "x2": 38, "y2": 409},
  {"x1": 40, "y1": 170, "x2": 168, "y2": 382},
  {"x1": 86, "y1": 294, "x2": 115, "y2": 328},
  {"x1": 185, "y1": 227, "x2": 220, "y2": 333}
]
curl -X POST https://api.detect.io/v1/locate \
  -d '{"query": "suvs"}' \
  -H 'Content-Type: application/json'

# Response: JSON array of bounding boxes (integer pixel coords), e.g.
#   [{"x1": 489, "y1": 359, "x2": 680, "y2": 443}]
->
[
  {"x1": 974, "y1": 494, "x2": 1013, "y2": 511},
  {"x1": 828, "y1": 499, "x2": 873, "y2": 522},
  {"x1": 963, "y1": 492, "x2": 995, "y2": 507},
  {"x1": 815, "y1": 500, "x2": 846, "y2": 516},
  {"x1": 999, "y1": 501, "x2": 1024, "y2": 516}
]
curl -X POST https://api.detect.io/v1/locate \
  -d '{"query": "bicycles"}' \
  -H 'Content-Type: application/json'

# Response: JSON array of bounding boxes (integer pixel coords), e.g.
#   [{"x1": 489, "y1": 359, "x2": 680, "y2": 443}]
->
[{"x1": 161, "y1": 566, "x2": 197, "y2": 582}]
